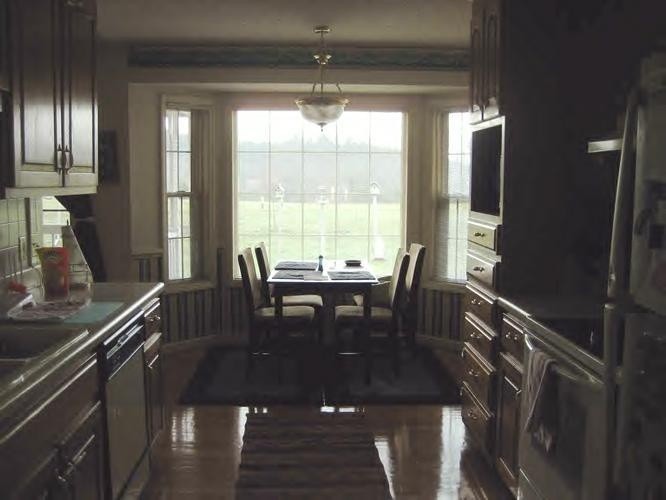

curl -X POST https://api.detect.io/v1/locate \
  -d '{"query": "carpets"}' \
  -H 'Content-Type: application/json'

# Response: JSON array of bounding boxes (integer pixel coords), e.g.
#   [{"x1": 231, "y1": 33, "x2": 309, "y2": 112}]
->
[
  {"x1": 236, "y1": 413, "x2": 392, "y2": 500},
  {"x1": 178, "y1": 345, "x2": 462, "y2": 406}
]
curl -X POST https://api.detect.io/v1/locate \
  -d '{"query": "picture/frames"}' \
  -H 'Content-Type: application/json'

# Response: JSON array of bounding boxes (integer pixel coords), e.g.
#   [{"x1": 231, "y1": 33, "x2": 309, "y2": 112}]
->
[{"x1": 98, "y1": 130, "x2": 118, "y2": 184}]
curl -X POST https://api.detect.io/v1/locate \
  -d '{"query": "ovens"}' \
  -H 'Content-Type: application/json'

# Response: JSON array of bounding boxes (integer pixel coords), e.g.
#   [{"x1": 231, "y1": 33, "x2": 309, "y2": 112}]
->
[
  {"x1": 96, "y1": 324, "x2": 153, "y2": 500},
  {"x1": 515, "y1": 325, "x2": 614, "y2": 500}
]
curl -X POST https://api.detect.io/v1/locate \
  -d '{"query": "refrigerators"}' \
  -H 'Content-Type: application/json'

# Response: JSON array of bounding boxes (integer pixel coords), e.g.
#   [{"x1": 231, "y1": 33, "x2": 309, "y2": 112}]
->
[{"x1": 606, "y1": 74, "x2": 666, "y2": 500}]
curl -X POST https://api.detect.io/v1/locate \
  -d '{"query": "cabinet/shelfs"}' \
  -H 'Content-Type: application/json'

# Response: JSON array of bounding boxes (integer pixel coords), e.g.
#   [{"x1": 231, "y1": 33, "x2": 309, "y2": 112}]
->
[
  {"x1": 0, "y1": 0, "x2": 98, "y2": 199},
  {"x1": 497, "y1": 306, "x2": 526, "y2": 499},
  {"x1": 0, "y1": 353, "x2": 106, "y2": 500},
  {"x1": 468, "y1": 0, "x2": 519, "y2": 124},
  {"x1": 139, "y1": 303, "x2": 165, "y2": 473},
  {"x1": 460, "y1": 291, "x2": 498, "y2": 467}
]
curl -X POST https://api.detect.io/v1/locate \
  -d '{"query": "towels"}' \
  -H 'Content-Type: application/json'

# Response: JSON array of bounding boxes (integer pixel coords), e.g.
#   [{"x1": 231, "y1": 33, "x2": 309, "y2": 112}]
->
[{"x1": 524, "y1": 348, "x2": 556, "y2": 452}]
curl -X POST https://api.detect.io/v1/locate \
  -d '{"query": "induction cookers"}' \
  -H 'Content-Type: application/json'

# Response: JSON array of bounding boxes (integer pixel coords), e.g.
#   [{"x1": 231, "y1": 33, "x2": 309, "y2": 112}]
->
[{"x1": 535, "y1": 318, "x2": 606, "y2": 367}]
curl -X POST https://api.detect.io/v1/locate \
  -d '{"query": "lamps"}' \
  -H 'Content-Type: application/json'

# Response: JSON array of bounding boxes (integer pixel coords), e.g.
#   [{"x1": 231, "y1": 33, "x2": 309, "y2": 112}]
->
[{"x1": 296, "y1": 26, "x2": 348, "y2": 131}]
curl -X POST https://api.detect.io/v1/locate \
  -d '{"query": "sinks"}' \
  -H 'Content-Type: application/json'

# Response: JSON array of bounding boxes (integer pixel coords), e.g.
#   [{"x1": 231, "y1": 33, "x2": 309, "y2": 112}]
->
[{"x1": 0, "y1": 325, "x2": 86, "y2": 359}]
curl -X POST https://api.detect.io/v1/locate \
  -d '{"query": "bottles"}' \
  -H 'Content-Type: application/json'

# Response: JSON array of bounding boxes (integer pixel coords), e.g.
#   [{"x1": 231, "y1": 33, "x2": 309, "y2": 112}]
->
[{"x1": 318, "y1": 255, "x2": 324, "y2": 273}]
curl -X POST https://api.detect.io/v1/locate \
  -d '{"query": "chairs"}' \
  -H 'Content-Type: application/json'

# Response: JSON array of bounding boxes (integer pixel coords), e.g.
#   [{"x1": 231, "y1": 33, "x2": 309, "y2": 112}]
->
[{"x1": 238, "y1": 243, "x2": 425, "y2": 385}]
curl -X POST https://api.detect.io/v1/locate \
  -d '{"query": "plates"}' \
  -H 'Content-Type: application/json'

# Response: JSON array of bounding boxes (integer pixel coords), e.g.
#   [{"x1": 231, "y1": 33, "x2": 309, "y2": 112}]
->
[{"x1": 345, "y1": 260, "x2": 360, "y2": 266}]
